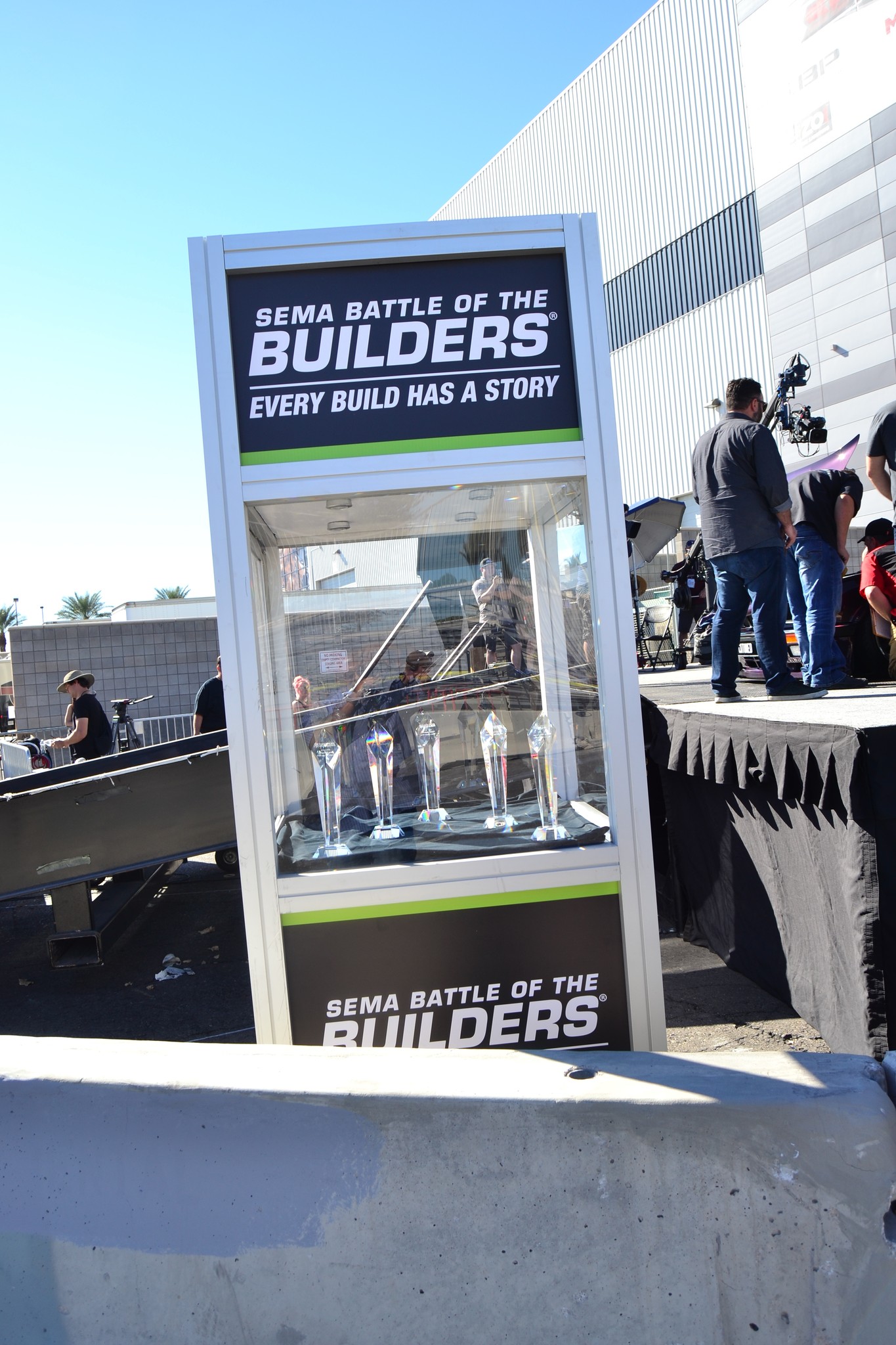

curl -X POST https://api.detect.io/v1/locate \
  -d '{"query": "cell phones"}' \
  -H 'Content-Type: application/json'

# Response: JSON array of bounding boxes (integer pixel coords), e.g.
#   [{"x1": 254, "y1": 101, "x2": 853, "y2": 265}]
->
[{"x1": 495, "y1": 569, "x2": 502, "y2": 578}]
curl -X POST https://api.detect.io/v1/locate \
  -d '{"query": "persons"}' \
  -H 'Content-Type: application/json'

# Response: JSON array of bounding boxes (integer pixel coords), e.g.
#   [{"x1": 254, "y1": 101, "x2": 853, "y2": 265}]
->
[
  {"x1": 692, "y1": 377, "x2": 828, "y2": 703},
  {"x1": 638, "y1": 693, "x2": 671, "y2": 933},
  {"x1": 292, "y1": 676, "x2": 310, "y2": 716},
  {"x1": 389, "y1": 651, "x2": 436, "y2": 708},
  {"x1": 661, "y1": 540, "x2": 707, "y2": 666},
  {"x1": 857, "y1": 518, "x2": 896, "y2": 679},
  {"x1": 508, "y1": 557, "x2": 538, "y2": 676},
  {"x1": 576, "y1": 551, "x2": 596, "y2": 671},
  {"x1": 303, "y1": 648, "x2": 426, "y2": 865},
  {"x1": 472, "y1": 557, "x2": 522, "y2": 672},
  {"x1": 64, "y1": 696, "x2": 75, "y2": 762},
  {"x1": 192, "y1": 656, "x2": 227, "y2": 736},
  {"x1": 865, "y1": 400, "x2": 896, "y2": 527},
  {"x1": 783, "y1": 468, "x2": 869, "y2": 692},
  {"x1": 50, "y1": 670, "x2": 113, "y2": 764}
]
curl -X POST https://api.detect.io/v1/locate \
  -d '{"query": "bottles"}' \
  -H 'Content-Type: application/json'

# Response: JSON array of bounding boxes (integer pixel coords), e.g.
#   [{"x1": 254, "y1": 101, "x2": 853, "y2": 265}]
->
[{"x1": 43, "y1": 739, "x2": 68, "y2": 748}]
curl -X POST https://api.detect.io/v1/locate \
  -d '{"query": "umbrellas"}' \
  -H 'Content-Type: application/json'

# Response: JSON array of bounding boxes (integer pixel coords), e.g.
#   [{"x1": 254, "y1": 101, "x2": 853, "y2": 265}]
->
[{"x1": 623, "y1": 496, "x2": 686, "y2": 671}]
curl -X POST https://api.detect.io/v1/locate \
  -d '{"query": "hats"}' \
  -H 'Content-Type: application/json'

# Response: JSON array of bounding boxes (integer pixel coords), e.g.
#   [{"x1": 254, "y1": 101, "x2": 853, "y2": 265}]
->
[
  {"x1": 480, "y1": 557, "x2": 497, "y2": 568},
  {"x1": 857, "y1": 518, "x2": 894, "y2": 543},
  {"x1": 685, "y1": 540, "x2": 695, "y2": 547},
  {"x1": 418, "y1": 650, "x2": 434, "y2": 657},
  {"x1": 406, "y1": 650, "x2": 437, "y2": 667},
  {"x1": 57, "y1": 670, "x2": 95, "y2": 693}
]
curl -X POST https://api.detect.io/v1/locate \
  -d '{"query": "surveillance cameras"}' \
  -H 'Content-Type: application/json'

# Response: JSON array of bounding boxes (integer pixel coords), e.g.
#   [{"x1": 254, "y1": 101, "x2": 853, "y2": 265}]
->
[{"x1": 793, "y1": 409, "x2": 828, "y2": 444}]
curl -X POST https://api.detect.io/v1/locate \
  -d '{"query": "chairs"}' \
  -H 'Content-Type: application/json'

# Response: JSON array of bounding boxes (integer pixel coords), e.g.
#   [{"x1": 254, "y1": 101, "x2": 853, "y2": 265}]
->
[{"x1": 635, "y1": 604, "x2": 677, "y2": 672}]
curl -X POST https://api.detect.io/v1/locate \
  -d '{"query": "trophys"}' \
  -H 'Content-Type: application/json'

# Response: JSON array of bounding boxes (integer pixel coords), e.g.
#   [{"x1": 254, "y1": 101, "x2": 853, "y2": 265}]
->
[
  {"x1": 478, "y1": 710, "x2": 521, "y2": 830},
  {"x1": 409, "y1": 705, "x2": 452, "y2": 823},
  {"x1": 366, "y1": 721, "x2": 406, "y2": 840},
  {"x1": 528, "y1": 708, "x2": 575, "y2": 842},
  {"x1": 310, "y1": 728, "x2": 352, "y2": 860}
]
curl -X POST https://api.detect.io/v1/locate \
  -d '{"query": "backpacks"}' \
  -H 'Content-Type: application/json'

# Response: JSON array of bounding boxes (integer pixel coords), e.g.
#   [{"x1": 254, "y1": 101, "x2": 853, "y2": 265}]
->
[{"x1": 90, "y1": 710, "x2": 113, "y2": 756}]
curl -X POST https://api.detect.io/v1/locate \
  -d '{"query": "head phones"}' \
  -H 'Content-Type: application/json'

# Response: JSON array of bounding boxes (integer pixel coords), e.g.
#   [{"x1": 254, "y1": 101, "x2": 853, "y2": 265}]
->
[{"x1": 411, "y1": 652, "x2": 426, "y2": 672}]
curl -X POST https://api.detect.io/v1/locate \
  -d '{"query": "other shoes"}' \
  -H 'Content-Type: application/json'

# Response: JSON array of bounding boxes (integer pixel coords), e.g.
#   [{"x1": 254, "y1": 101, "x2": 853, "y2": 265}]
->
[
  {"x1": 816, "y1": 675, "x2": 869, "y2": 691},
  {"x1": 525, "y1": 667, "x2": 539, "y2": 675}
]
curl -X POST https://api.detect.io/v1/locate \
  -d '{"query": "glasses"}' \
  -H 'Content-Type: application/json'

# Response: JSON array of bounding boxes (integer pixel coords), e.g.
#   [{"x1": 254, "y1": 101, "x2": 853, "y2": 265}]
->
[{"x1": 750, "y1": 397, "x2": 768, "y2": 413}]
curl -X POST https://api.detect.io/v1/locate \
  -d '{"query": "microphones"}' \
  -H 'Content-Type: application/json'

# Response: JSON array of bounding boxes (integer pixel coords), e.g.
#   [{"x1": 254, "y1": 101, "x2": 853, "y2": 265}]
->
[{"x1": 106, "y1": 716, "x2": 142, "y2": 755}]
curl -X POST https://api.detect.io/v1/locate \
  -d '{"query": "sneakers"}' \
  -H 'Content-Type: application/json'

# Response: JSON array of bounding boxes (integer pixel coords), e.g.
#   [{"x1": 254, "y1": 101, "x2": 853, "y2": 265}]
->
[
  {"x1": 714, "y1": 689, "x2": 742, "y2": 703},
  {"x1": 767, "y1": 678, "x2": 829, "y2": 701}
]
